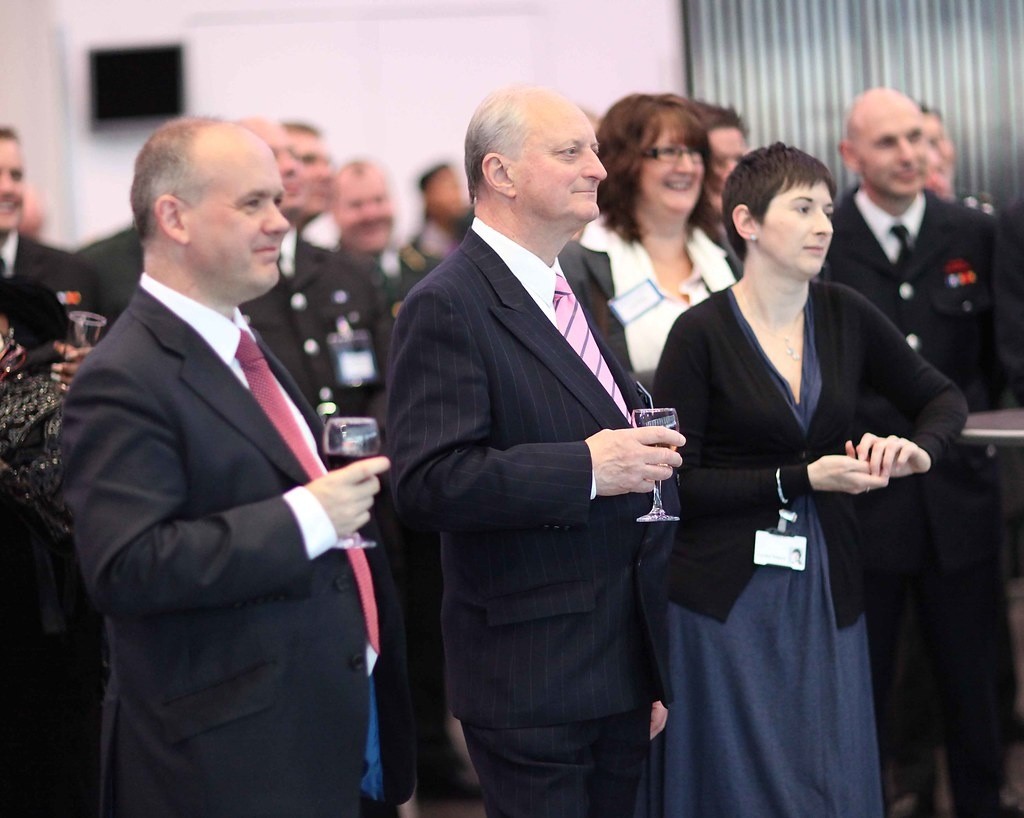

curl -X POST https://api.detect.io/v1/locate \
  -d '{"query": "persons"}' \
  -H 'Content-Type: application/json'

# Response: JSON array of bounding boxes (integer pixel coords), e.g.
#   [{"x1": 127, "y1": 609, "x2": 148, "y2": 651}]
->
[
  {"x1": 920, "y1": 108, "x2": 997, "y2": 216},
  {"x1": 995, "y1": 197, "x2": 1024, "y2": 408},
  {"x1": 638, "y1": 141, "x2": 968, "y2": 818},
  {"x1": 0, "y1": 120, "x2": 465, "y2": 446},
  {"x1": 818, "y1": 86, "x2": 1023, "y2": 818},
  {"x1": 61, "y1": 117, "x2": 418, "y2": 818},
  {"x1": 581, "y1": 93, "x2": 742, "y2": 395},
  {"x1": 382, "y1": 83, "x2": 685, "y2": 817},
  {"x1": 696, "y1": 100, "x2": 750, "y2": 281}
]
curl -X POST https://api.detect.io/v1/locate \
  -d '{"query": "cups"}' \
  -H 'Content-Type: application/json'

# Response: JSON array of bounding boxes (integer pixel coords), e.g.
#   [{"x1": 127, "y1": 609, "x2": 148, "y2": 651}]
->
[{"x1": 61, "y1": 311, "x2": 108, "y2": 366}]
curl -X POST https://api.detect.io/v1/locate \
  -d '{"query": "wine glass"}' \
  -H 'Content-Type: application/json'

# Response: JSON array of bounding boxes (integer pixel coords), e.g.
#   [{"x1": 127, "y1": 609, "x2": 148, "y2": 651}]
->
[
  {"x1": 322, "y1": 416, "x2": 384, "y2": 550},
  {"x1": 631, "y1": 406, "x2": 681, "y2": 523}
]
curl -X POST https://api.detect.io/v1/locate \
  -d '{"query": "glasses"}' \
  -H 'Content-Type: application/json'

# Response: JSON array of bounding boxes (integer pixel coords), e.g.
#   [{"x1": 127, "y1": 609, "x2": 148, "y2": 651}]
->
[{"x1": 639, "y1": 143, "x2": 707, "y2": 164}]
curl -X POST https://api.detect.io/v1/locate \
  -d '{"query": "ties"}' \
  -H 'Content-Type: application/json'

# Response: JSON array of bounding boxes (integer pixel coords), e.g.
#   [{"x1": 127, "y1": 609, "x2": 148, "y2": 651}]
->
[
  {"x1": 553, "y1": 273, "x2": 632, "y2": 425},
  {"x1": 890, "y1": 223, "x2": 912, "y2": 271},
  {"x1": 236, "y1": 327, "x2": 381, "y2": 656}
]
